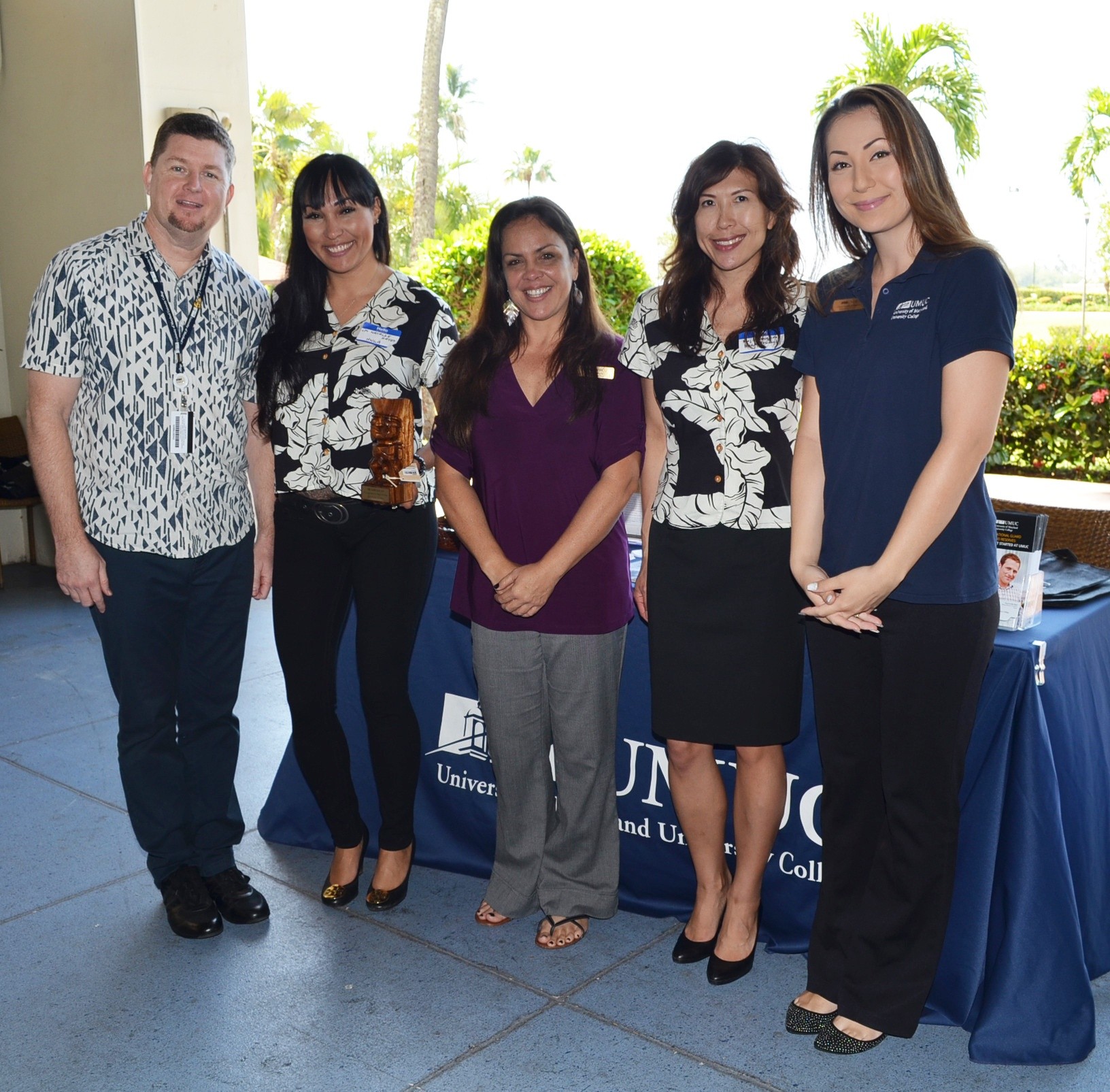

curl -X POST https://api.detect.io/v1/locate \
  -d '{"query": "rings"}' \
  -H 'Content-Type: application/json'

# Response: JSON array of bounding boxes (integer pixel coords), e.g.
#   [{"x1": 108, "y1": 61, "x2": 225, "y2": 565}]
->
[{"x1": 854, "y1": 613, "x2": 859, "y2": 618}]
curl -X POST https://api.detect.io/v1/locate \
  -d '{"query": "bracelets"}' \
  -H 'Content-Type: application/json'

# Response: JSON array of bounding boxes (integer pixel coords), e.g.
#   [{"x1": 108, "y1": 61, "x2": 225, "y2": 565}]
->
[{"x1": 413, "y1": 453, "x2": 427, "y2": 474}]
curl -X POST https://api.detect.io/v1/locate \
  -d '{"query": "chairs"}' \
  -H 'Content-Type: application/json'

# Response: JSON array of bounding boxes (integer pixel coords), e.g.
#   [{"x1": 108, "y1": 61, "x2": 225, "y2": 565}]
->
[
  {"x1": 991, "y1": 498, "x2": 1110, "y2": 572},
  {"x1": 0, "y1": 415, "x2": 45, "y2": 587}
]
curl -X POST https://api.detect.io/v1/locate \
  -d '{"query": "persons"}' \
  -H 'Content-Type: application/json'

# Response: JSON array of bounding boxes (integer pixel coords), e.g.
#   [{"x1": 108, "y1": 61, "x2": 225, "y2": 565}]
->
[
  {"x1": 246, "y1": 149, "x2": 456, "y2": 907},
  {"x1": 996, "y1": 553, "x2": 1024, "y2": 627},
  {"x1": 18, "y1": 113, "x2": 277, "y2": 937},
  {"x1": 615, "y1": 140, "x2": 812, "y2": 984},
  {"x1": 421, "y1": 199, "x2": 646, "y2": 952},
  {"x1": 775, "y1": 84, "x2": 1020, "y2": 1055}
]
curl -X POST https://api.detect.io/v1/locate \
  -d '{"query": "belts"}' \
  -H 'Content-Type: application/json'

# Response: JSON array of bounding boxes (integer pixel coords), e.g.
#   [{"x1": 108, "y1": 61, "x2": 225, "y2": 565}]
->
[{"x1": 278, "y1": 492, "x2": 378, "y2": 526}]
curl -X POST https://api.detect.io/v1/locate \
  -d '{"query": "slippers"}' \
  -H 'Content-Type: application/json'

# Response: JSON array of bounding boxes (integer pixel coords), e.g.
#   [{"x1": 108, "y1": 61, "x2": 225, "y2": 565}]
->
[
  {"x1": 475, "y1": 900, "x2": 512, "y2": 925},
  {"x1": 536, "y1": 915, "x2": 588, "y2": 948}
]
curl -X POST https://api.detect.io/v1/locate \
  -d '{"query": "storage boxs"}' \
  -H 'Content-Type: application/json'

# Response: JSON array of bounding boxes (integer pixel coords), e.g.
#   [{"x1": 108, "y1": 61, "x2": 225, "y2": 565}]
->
[{"x1": 996, "y1": 570, "x2": 1044, "y2": 631}]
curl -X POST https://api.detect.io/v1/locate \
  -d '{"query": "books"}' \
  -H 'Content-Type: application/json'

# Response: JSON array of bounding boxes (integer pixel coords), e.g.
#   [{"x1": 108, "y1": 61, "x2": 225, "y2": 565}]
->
[{"x1": 993, "y1": 508, "x2": 1050, "y2": 630}]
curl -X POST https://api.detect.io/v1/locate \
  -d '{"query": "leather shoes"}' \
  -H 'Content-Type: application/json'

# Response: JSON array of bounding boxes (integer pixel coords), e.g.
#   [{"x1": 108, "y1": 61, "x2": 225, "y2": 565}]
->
[
  {"x1": 160, "y1": 872, "x2": 224, "y2": 939},
  {"x1": 205, "y1": 867, "x2": 270, "y2": 924}
]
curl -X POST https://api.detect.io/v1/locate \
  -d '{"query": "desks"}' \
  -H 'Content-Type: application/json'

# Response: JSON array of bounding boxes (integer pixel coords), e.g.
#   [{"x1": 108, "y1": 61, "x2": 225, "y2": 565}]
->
[{"x1": 258, "y1": 549, "x2": 1109, "y2": 1064}]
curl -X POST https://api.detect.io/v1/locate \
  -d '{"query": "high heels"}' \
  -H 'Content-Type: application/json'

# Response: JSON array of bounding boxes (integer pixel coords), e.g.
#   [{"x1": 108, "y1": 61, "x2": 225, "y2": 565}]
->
[
  {"x1": 366, "y1": 834, "x2": 416, "y2": 911},
  {"x1": 672, "y1": 898, "x2": 725, "y2": 964},
  {"x1": 708, "y1": 900, "x2": 761, "y2": 983},
  {"x1": 321, "y1": 822, "x2": 370, "y2": 907}
]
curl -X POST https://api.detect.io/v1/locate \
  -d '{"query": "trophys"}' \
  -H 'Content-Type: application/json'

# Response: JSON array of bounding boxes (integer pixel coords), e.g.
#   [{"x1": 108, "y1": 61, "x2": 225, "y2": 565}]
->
[{"x1": 359, "y1": 395, "x2": 415, "y2": 506}]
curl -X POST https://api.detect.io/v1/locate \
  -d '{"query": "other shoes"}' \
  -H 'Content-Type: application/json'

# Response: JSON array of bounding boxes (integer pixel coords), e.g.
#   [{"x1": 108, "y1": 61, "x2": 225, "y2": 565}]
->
[
  {"x1": 785, "y1": 998, "x2": 841, "y2": 1033},
  {"x1": 813, "y1": 1017, "x2": 889, "y2": 1053}
]
